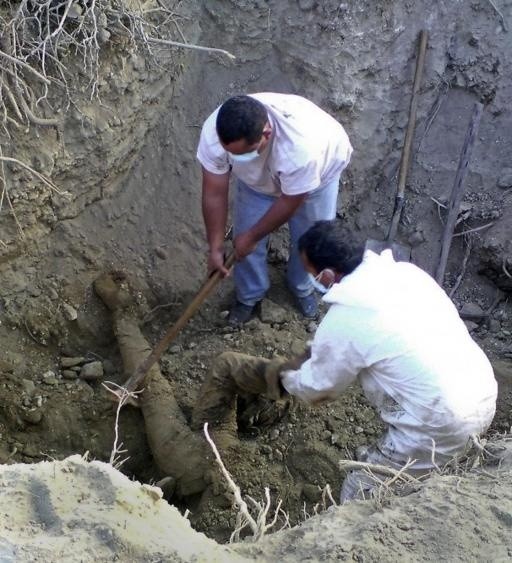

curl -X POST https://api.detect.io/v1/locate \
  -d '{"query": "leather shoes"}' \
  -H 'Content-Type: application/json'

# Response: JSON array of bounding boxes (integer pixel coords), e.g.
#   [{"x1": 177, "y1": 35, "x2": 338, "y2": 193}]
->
[
  {"x1": 228, "y1": 300, "x2": 254, "y2": 329},
  {"x1": 295, "y1": 292, "x2": 318, "y2": 318}
]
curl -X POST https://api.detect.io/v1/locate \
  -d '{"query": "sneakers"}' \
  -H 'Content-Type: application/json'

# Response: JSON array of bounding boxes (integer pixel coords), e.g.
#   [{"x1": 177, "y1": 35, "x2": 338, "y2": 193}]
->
[{"x1": 355, "y1": 444, "x2": 368, "y2": 459}]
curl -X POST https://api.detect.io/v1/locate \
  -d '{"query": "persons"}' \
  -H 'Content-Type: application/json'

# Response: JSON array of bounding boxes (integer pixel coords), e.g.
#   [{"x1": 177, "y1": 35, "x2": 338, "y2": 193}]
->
[
  {"x1": 196, "y1": 92, "x2": 354, "y2": 327},
  {"x1": 278, "y1": 220, "x2": 499, "y2": 504},
  {"x1": 94, "y1": 274, "x2": 312, "y2": 497}
]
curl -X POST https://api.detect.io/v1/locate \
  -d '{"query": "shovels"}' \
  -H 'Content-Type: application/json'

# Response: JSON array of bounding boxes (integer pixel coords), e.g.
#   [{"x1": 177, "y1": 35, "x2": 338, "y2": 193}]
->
[{"x1": 363, "y1": 28, "x2": 429, "y2": 263}]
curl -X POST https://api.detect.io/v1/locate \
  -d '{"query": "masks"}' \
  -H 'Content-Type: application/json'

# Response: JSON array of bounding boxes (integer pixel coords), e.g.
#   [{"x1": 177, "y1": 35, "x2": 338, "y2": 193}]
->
[
  {"x1": 224, "y1": 150, "x2": 260, "y2": 162},
  {"x1": 307, "y1": 272, "x2": 328, "y2": 294}
]
